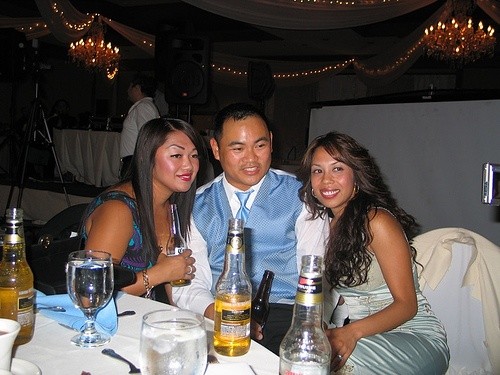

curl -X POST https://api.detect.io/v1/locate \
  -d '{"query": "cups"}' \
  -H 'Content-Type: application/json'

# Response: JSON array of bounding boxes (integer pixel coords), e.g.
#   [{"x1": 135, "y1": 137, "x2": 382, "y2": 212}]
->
[
  {"x1": 139, "y1": 309, "x2": 209, "y2": 375},
  {"x1": 0, "y1": 317, "x2": 21, "y2": 372}
]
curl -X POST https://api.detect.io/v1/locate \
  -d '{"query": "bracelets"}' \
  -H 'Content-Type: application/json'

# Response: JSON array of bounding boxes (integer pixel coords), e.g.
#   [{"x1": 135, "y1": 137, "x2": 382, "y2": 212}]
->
[{"x1": 142, "y1": 270, "x2": 149, "y2": 293}]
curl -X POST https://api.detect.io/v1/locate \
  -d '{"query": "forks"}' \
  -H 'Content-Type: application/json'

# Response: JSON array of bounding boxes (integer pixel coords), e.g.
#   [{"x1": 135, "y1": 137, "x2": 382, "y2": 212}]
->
[{"x1": 102, "y1": 348, "x2": 141, "y2": 375}]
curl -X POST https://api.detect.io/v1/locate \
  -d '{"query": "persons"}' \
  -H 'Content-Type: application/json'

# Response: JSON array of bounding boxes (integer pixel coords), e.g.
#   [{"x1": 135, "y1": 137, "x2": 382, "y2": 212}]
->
[
  {"x1": 119, "y1": 74, "x2": 160, "y2": 180},
  {"x1": 295, "y1": 131, "x2": 450, "y2": 375},
  {"x1": 171, "y1": 104, "x2": 329, "y2": 356},
  {"x1": 77, "y1": 117, "x2": 202, "y2": 306}
]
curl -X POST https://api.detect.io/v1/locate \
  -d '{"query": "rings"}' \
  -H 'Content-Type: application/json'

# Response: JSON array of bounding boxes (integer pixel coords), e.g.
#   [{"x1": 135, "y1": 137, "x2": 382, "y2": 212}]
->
[{"x1": 338, "y1": 354, "x2": 342, "y2": 360}]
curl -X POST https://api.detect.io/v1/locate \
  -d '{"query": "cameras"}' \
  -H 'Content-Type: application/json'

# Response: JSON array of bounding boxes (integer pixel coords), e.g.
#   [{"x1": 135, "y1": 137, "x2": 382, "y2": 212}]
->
[{"x1": 480, "y1": 161, "x2": 500, "y2": 206}]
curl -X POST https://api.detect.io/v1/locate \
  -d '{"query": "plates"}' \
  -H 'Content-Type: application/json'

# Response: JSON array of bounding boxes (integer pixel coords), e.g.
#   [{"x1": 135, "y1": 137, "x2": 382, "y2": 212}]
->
[{"x1": 8, "y1": 358, "x2": 42, "y2": 375}]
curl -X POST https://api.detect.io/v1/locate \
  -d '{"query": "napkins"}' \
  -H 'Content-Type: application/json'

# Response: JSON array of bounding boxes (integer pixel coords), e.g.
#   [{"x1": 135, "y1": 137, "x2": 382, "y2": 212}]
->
[{"x1": 36, "y1": 290, "x2": 117, "y2": 335}]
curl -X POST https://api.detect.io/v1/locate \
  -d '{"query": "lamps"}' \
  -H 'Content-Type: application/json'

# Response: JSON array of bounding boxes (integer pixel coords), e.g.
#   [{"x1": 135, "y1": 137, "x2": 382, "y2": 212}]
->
[
  {"x1": 418, "y1": 0, "x2": 496, "y2": 65},
  {"x1": 67, "y1": 17, "x2": 121, "y2": 79}
]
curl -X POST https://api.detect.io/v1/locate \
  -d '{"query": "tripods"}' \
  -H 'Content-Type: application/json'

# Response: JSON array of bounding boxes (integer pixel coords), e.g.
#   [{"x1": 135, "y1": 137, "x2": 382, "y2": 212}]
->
[{"x1": 1, "y1": 74, "x2": 71, "y2": 217}]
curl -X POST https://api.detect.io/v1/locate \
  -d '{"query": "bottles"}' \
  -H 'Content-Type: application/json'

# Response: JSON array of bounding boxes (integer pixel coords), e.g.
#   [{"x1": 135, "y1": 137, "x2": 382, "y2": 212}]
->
[
  {"x1": 250, "y1": 270, "x2": 274, "y2": 343},
  {"x1": 166, "y1": 203, "x2": 191, "y2": 288},
  {"x1": 0, "y1": 208, "x2": 35, "y2": 346},
  {"x1": 213, "y1": 217, "x2": 253, "y2": 357},
  {"x1": 278, "y1": 255, "x2": 332, "y2": 375}
]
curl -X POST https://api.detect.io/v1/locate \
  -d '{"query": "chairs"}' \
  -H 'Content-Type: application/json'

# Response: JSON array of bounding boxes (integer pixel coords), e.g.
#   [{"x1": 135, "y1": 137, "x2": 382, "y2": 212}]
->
[{"x1": 411, "y1": 228, "x2": 500, "y2": 375}]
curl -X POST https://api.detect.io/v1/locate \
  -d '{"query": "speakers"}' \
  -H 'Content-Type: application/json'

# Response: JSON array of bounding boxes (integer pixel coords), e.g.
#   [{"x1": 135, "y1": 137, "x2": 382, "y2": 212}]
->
[
  {"x1": 154, "y1": 31, "x2": 208, "y2": 104},
  {"x1": 0, "y1": 27, "x2": 26, "y2": 84}
]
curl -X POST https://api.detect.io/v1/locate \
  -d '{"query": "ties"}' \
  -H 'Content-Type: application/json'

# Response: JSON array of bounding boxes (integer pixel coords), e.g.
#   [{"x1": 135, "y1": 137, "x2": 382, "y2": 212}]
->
[{"x1": 235, "y1": 190, "x2": 254, "y2": 227}]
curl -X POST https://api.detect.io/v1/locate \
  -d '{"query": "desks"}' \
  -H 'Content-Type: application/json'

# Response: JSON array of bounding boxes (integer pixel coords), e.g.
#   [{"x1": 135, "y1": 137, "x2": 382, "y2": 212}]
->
[
  {"x1": 53, "y1": 127, "x2": 122, "y2": 188},
  {"x1": 11, "y1": 292, "x2": 281, "y2": 375}
]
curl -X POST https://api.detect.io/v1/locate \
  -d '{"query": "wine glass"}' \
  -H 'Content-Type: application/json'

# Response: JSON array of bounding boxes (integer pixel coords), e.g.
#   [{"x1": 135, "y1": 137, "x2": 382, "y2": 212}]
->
[{"x1": 65, "y1": 250, "x2": 115, "y2": 348}]
